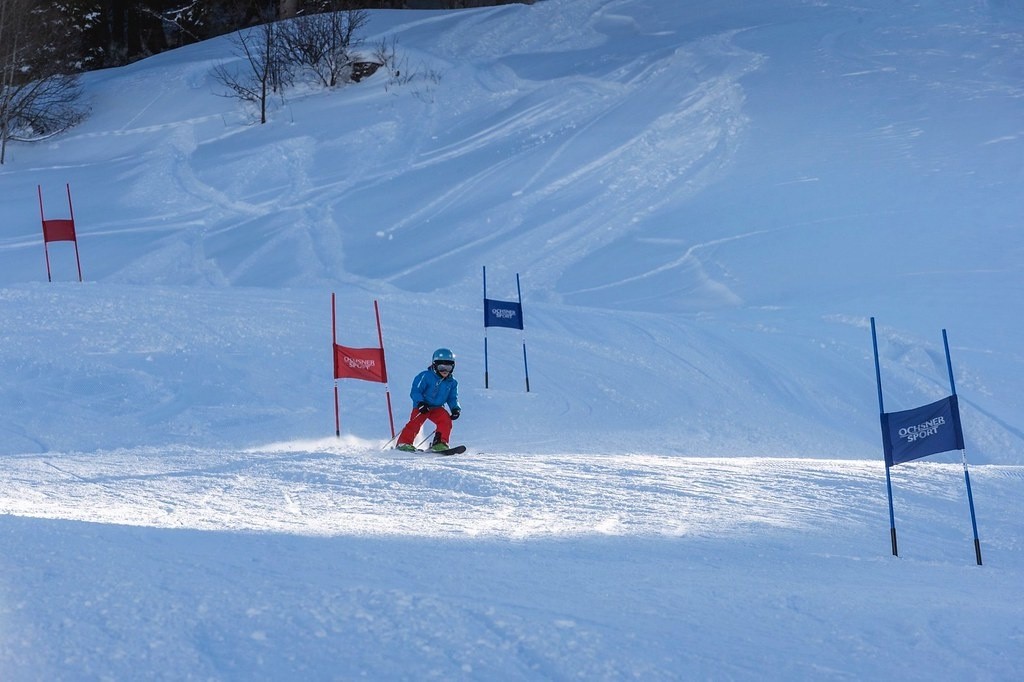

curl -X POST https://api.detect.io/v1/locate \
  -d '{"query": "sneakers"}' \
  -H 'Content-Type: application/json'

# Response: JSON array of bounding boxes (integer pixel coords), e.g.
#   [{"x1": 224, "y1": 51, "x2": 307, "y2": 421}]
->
[
  {"x1": 397, "y1": 443, "x2": 416, "y2": 450},
  {"x1": 429, "y1": 443, "x2": 449, "y2": 451}
]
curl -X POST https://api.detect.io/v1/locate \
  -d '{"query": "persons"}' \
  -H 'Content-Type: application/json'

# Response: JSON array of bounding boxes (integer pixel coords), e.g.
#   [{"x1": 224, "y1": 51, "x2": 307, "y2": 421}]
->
[{"x1": 396, "y1": 348, "x2": 461, "y2": 453}]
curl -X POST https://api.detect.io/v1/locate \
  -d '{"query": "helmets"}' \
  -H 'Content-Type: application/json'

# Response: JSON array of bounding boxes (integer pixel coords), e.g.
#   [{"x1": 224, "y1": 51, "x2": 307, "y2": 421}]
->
[{"x1": 433, "y1": 348, "x2": 455, "y2": 362}]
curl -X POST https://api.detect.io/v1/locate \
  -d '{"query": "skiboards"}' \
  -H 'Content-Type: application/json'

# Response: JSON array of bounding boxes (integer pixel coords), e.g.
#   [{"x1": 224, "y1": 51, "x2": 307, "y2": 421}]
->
[{"x1": 397, "y1": 444, "x2": 466, "y2": 455}]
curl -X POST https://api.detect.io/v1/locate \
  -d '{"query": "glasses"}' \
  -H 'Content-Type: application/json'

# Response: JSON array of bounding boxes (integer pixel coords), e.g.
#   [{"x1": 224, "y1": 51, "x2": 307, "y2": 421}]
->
[{"x1": 434, "y1": 363, "x2": 455, "y2": 372}]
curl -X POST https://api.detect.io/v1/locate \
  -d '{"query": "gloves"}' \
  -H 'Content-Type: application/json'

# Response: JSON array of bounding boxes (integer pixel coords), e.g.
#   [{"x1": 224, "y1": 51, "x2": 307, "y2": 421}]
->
[
  {"x1": 418, "y1": 401, "x2": 430, "y2": 414},
  {"x1": 450, "y1": 410, "x2": 460, "y2": 420}
]
